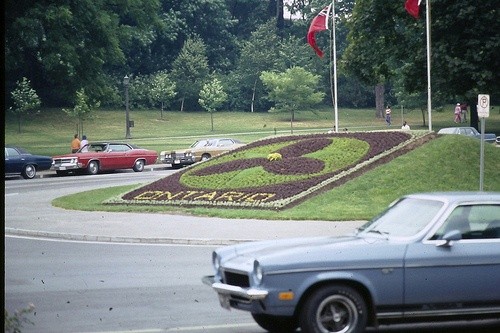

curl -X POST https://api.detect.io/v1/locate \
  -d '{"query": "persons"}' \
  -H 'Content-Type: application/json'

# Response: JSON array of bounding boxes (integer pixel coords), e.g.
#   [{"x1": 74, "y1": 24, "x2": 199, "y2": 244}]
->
[
  {"x1": 385, "y1": 105, "x2": 391, "y2": 126},
  {"x1": 72, "y1": 134, "x2": 81, "y2": 153},
  {"x1": 455, "y1": 103, "x2": 462, "y2": 124},
  {"x1": 80, "y1": 135, "x2": 88, "y2": 152},
  {"x1": 401, "y1": 121, "x2": 410, "y2": 130},
  {"x1": 461, "y1": 103, "x2": 467, "y2": 119}
]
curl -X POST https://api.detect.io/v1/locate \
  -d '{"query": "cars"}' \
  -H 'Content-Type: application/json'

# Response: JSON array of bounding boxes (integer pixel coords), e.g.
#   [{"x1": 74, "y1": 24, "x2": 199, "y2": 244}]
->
[
  {"x1": 48, "y1": 142, "x2": 159, "y2": 177},
  {"x1": 437, "y1": 127, "x2": 500, "y2": 145},
  {"x1": 200, "y1": 191, "x2": 500, "y2": 333},
  {"x1": 4, "y1": 145, "x2": 54, "y2": 180},
  {"x1": 160, "y1": 138, "x2": 247, "y2": 170}
]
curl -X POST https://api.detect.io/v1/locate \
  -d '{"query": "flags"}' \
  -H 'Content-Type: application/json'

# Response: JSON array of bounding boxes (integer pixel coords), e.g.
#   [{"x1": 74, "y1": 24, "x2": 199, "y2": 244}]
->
[
  {"x1": 405, "y1": 0, "x2": 422, "y2": 19},
  {"x1": 307, "y1": 3, "x2": 331, "y2": 57}
]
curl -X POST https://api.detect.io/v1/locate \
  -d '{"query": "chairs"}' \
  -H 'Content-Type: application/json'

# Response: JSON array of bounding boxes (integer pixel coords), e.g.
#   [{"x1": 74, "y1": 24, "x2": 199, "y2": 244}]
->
[
  {"x1": 484, "y1": 219, "x2": 500, "y2": 237},
  {"x1": 439, "y1": 215, "x2": 471, "y2": 238}
]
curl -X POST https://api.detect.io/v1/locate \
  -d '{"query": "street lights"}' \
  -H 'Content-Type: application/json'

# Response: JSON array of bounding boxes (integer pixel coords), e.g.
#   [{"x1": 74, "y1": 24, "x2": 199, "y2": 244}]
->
[{"x1": 122, "y1": 75, "x2": 133, "y2": 139}]
